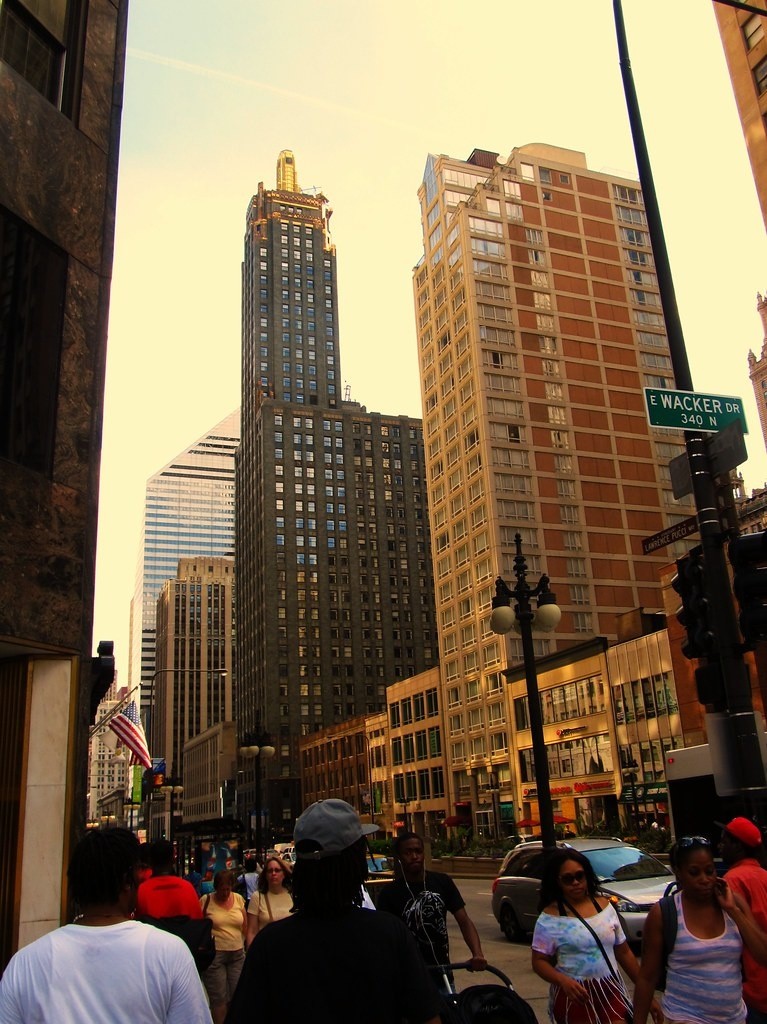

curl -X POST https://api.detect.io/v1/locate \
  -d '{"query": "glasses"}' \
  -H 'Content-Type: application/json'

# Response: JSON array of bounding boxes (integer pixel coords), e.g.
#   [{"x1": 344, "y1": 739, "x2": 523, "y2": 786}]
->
[
  {"x1": 719, "y1": 839, "x2": 730, "y2": 847},
  {"x1": 267, "y1": 868, "x2": 283, "y2": 872},
  {"x1": 558, "y1": 870, "x2": 585, "y2": 885},
  {"x1": 674, "y1": 836, "x2": 711, "y2": 847}
]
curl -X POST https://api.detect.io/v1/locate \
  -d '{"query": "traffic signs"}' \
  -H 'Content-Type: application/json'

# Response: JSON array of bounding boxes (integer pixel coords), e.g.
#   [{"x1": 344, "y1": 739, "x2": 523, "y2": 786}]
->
[
  {"x1": 643, "y1": 388, "x2": 749, "y2": 434},
  {"x1": 640, "y1": 515, "x2": 701, "y2": 557}
]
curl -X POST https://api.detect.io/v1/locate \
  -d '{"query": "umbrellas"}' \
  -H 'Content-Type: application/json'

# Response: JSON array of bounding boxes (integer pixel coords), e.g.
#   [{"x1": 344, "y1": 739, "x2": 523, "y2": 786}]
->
[
  {"x1": 512, "y1": 819, "x2": 542, "y2": 835},
  {"x1": 553, "y1": 814, "x2": 583, "y2": 838}
]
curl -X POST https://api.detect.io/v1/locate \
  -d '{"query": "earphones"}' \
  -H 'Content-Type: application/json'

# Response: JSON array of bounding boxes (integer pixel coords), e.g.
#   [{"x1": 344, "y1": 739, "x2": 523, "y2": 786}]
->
[{"x1": 397, "y1": 858, "x2": 401, "y2": 863}]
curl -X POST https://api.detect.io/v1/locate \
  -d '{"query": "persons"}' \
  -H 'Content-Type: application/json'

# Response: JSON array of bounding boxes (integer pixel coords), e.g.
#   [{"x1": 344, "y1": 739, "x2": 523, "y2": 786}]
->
[
  {"x1": 224, "y1": 798, "x2": 440, "y2": 1024},
  {"x1": 531, "y1": 848, "x2": 664, "y2": 1024},
  {"x1": 130, "y1": 842, "x2": 296, "y2": 1024},
  {"x1": 0, "y1": 828, "x2": 214, "y2": 1024},
  {"x1": 632, "y1": 835, "x2": 767, "y2": 1024},
  {"x1": 712, "y1": 816, "x2": 767, "y2": 1024},
  {"x1": 374, "y1": 833, "x2": 487, "y2": 996}
]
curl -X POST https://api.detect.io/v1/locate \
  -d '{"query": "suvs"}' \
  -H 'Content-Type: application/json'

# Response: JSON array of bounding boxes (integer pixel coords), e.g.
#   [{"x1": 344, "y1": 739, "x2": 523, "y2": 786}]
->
[{"x1": 492, "y1": 837, "x2": 676, "y2": 950}]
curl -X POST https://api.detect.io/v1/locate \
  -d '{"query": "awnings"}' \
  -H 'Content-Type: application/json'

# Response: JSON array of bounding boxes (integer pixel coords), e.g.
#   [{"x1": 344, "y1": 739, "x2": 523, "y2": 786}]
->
[{"x1": 441, "y1": 816, "x2": 473, "y2": 829}]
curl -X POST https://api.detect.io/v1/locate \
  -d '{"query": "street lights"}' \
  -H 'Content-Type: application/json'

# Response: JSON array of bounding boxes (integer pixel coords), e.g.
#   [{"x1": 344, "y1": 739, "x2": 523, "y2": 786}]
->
[
  {"x1": 327, "y1": 735, "x2": 375, "y2": 841},
  {"x1": 160, "y1": 776, "x2": 185, "y2": 874},
  {"x1": 239, "y1": 732, "x2": 275, "y2": 863},
  {"x1": 621, "y1": 760, "x2": 641, "y2": 835},
  {"x1": 144, "y1": 668, "x2": 230, "y2": 843},
  {"x1": 100, "y1": 811, "x2": 116, "y2": 828},
  {"x1": 488, "y1": 575, "x2": 563, "y2": 851},
  {"x1": 123, "y1": 799, "x2": 141, "y2": 832},
  {"x1": 398, "y1": 797, "x2": 410, "y2": 832}
]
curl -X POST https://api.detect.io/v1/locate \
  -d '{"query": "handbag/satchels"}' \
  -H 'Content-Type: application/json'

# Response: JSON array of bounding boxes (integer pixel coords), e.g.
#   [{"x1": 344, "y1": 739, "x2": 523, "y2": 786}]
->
[
  {"x1": 628, "y1": 1007, "x2": 634, "y2": 1024},
  {"x1": 197, "y1": 929, "x2": 216, "y2": 970},
  {"x1": 237, "y1": 874, "x2": 248, "y2": 899}
]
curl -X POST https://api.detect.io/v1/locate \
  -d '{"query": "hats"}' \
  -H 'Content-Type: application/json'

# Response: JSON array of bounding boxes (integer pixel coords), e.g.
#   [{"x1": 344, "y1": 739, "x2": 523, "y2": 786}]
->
[
  {"x1": 293, "y1": 799, "x2": 379, "y2": 860},
  {"x1": 714, "y1": 817, "x2": 762, "y2": 847},
  {"x1": 67, "y1": 828, "x2": 150, "y2": 878}
]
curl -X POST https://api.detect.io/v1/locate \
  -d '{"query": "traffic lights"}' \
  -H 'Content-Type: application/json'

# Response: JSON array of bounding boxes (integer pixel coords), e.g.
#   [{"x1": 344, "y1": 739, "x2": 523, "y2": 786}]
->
[
  {"x1": 162, "y1": 829, "x2": 165, "y2": 839},
  {"x1": 730, "y1": 531, "x2": 767, "y2": 640},
  {"x1": 674, "y1": 561, "x2": 704, "y2": 662}
]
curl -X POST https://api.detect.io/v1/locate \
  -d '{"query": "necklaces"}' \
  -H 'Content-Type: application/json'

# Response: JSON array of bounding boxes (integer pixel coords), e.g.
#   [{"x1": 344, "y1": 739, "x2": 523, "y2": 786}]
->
[{"x1": 73, "y1": 912, "x2": 124, "y2": 924}]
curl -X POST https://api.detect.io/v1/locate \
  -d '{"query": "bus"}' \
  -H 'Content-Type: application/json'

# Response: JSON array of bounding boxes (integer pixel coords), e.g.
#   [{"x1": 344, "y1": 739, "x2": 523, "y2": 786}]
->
[{"x1": 666, "y1": 734, "x2": 767, "y2": 868}]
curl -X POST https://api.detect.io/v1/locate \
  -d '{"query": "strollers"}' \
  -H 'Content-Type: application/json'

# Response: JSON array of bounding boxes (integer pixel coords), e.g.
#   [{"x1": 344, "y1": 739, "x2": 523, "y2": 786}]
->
[{"x1": 429, "y1": 963, "x2": 538, "y2": 1024}]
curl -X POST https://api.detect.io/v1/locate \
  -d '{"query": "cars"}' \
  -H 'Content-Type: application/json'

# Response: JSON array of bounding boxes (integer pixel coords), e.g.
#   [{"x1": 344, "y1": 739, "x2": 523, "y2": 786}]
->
[
  {"x1": 175, "y1": 855, "x2": 189, "y2": 866},
  {"x1": 281, "y1": 852, "x2": 296, "y2": 864},
  {"x1": 243, "y1": 849, "x2": 256, "y2": 859},
  {"x1": 279, "y1": 847, "x2": 294, "y2": 857},
  {"x1": 261, "y1": 849, "x2": 279, "y2": 859},
  {"x1": 365, "y1": 854, "x2": 395, "y2": 879}
]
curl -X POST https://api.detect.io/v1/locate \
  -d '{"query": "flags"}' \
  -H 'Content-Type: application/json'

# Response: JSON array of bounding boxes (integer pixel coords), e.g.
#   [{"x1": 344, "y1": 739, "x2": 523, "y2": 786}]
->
[{"x1": 108, "y1": 701, "x2": 153, "y2": 770}]
